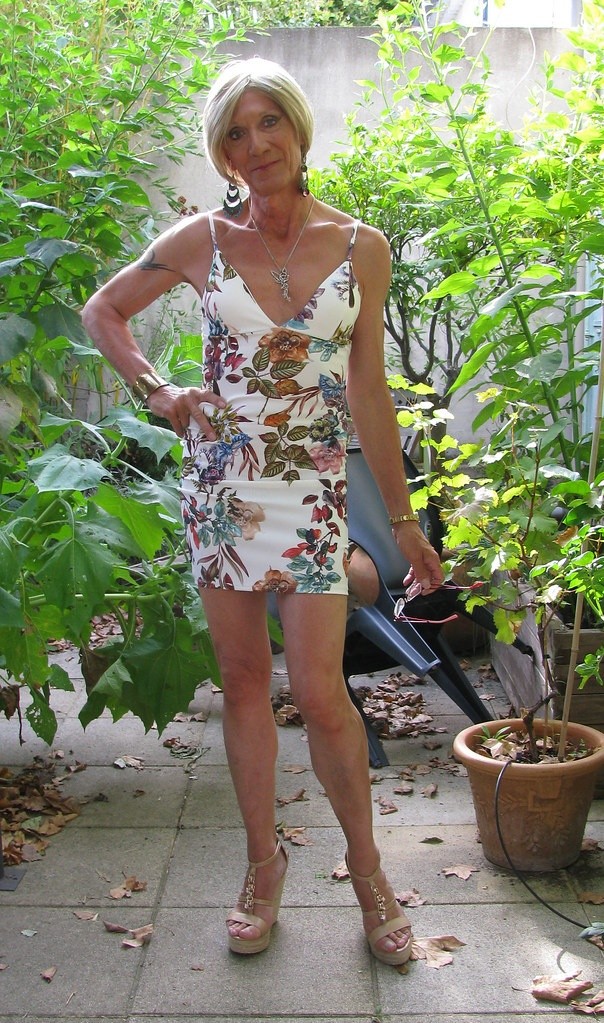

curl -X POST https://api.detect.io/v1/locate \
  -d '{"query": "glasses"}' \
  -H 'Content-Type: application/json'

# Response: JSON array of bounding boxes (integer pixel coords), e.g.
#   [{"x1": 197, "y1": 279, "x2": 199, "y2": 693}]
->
[{"x1": 392, "y1": 581, "x2": 482, "y2": 624}]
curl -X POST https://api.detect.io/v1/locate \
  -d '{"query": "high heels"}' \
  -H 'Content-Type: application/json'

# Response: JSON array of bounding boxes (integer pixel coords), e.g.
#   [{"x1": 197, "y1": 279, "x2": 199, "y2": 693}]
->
[
  {"x1": 224, "y1": 833, "x2": 288, "y2": 955},
  {"x1": 342, "y1": 842, "x2": 417, "y2": 965}
]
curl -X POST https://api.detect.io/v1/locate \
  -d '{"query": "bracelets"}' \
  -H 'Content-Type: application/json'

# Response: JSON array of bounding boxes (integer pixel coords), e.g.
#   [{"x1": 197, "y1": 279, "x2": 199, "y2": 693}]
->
[
  {"x1": 389, "y1": 514, "x2": 421, "y2": 525},
  {"x1": 132, "y1": 370, "x2": 170, "y2": 404}
]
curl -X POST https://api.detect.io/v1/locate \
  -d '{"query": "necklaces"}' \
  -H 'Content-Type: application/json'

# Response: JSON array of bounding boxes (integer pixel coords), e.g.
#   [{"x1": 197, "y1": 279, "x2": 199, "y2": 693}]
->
[{"x1": 247, "y1": 191, "x2": 315, "y2": 303}]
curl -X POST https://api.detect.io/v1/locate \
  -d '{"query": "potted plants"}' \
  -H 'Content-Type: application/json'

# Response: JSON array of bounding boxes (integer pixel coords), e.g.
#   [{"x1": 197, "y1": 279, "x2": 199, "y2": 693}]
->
[{"x1": 360, "y1": 0, "x2": 604, "y2": 877}]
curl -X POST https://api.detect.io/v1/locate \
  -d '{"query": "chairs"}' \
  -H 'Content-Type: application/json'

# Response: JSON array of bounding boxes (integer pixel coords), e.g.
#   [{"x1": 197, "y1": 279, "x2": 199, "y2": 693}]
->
[{"x1": 263, "y1": 440, "x2": 534, "y2": 769}]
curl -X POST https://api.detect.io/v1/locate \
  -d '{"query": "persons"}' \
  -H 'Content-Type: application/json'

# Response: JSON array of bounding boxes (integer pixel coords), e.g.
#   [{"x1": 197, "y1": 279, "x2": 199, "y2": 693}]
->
[{"x1": 81, "y1": 61, "x2": 444, "y2": 966}]
